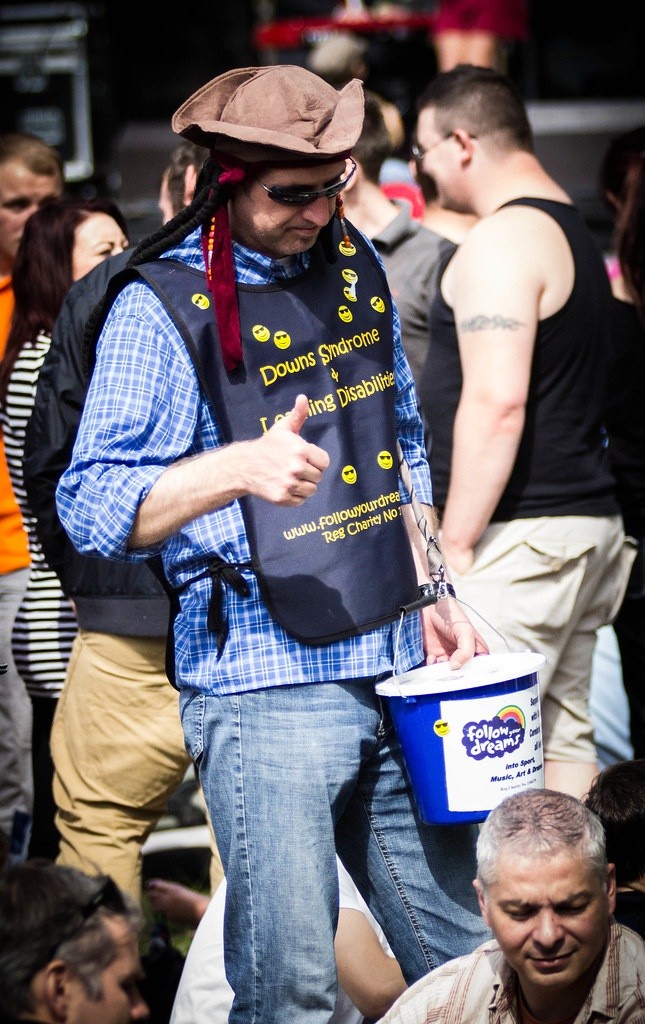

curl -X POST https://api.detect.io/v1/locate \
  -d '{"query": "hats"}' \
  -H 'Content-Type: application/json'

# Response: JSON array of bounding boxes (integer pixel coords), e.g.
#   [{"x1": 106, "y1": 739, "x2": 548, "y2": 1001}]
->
[{"x1": 171, "y1": 63, "x2": 365, "y2": 158}]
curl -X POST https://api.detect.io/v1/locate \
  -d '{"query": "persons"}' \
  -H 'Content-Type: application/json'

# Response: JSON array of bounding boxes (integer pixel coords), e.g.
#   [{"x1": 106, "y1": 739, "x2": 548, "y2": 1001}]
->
[
  {"x1": 55, "y1": 65, "x2": 497, "y2": 1023},
  {"x1": 312, "y1": 38, "x2": 645, "y2": 806},
  {"x1": 0, "y1": 131, "x2": 202, "y2": 932},
  {"x1": 372, "y1": 759, "x2": 645, "y2": 1024},
  {"x1": 0, "y1": 854, "x2": 408, "y2": 1024}
]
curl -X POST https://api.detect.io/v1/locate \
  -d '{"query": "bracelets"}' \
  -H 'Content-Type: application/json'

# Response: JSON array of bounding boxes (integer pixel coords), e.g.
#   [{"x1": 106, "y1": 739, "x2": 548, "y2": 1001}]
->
[{"x1": 419, "y1": 583, "x2": 456, "y2": 598}]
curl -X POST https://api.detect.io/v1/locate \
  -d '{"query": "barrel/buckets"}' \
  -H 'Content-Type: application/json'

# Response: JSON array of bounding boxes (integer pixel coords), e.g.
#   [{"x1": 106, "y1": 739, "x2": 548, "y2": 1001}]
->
[{"x1": 373, "y1": 654, "x2": 548, "y2": 826}]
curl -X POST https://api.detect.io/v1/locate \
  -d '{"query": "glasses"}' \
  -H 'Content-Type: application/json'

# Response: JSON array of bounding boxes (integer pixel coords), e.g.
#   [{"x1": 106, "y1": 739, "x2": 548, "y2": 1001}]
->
[
  {"x1": 411, "y1": 128, "x2": 478, "y2": 158},
  {"x1": 253, "y1": 160, "x2": 358, "y2": 205}
]
